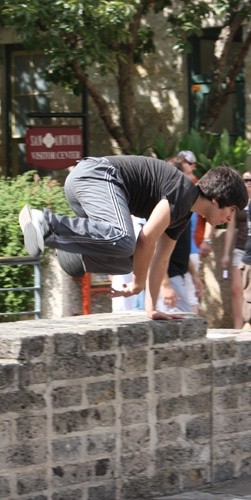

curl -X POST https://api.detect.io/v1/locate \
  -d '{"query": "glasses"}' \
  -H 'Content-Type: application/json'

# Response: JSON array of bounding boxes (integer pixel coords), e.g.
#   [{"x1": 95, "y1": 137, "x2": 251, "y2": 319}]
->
[{"x1": 243, "y1": 179, "x2": 251, "y2": 183}]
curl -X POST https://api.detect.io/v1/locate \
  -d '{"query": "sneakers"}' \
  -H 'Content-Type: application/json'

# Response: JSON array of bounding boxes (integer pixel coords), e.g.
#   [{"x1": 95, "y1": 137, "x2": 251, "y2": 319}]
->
[
  {"x1": 56, "y1": 248, "x2": 86, "y2": 278},
  {"x1": 18, "y1": 205, "x2": 52, "y2": 258}
]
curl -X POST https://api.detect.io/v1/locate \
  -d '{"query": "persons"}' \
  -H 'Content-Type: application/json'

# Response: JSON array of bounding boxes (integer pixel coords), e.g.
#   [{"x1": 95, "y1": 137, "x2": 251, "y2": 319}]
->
[
  {"x1": 128, "y1": 148, "x2": 251, "y2": 330},
  {"x1": 19, "y1": 155, "x2": 249, "y2": 320}
]
